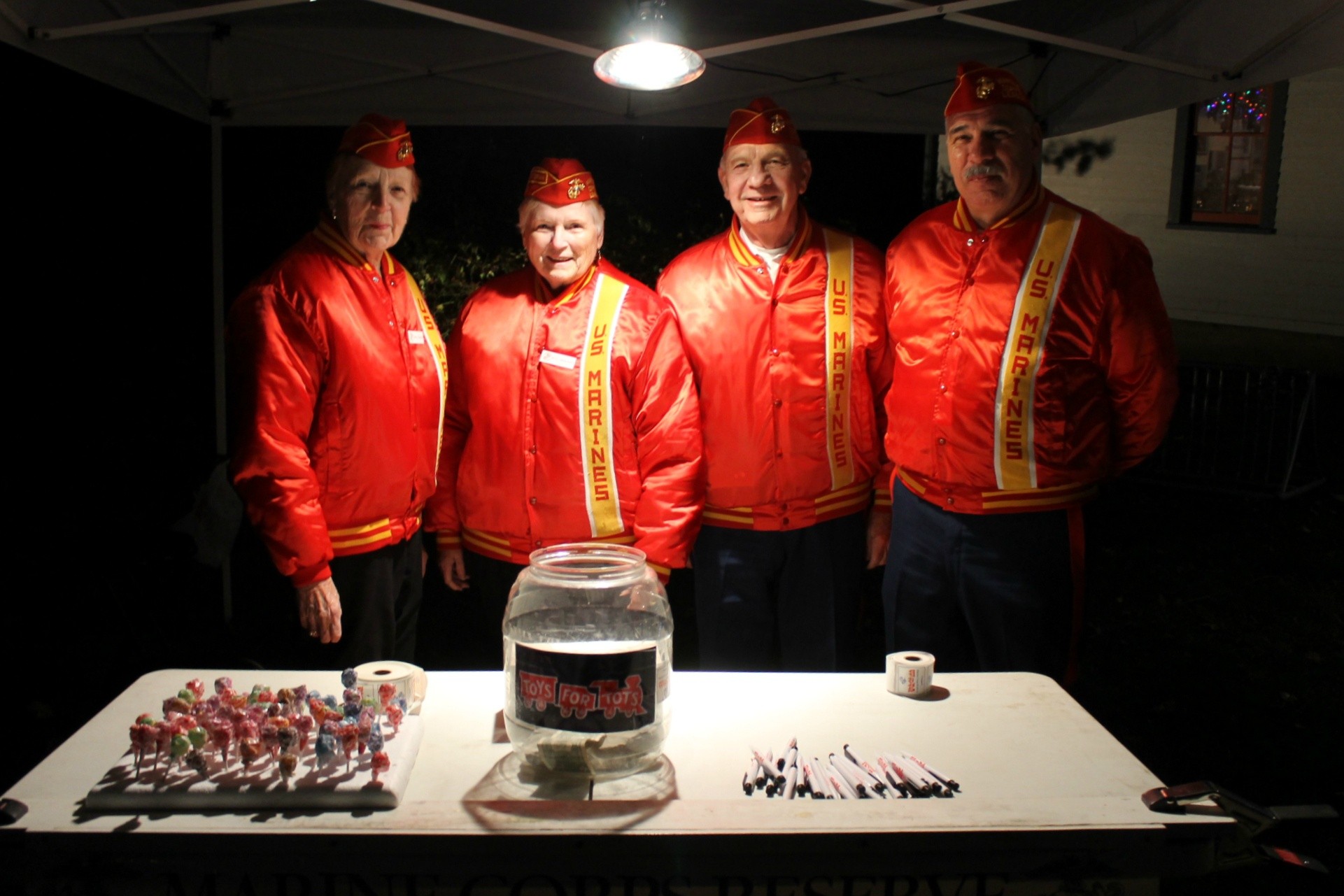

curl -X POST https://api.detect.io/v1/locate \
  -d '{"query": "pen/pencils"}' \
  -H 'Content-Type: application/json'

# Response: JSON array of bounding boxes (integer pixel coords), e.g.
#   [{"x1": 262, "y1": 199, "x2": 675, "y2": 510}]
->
[{"x1": 742, "y1": 736, "x2": 961, "y2": 802}]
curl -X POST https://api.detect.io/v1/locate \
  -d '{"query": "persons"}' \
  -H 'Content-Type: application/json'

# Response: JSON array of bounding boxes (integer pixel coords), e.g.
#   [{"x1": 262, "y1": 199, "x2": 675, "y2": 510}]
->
[
  {"x1": 883, "y1": 60, "x2": 1182, "y2": 693},
  {"x1": 420, "y1": 158, "x2": 707, "y2": 672},
  {"x1": 649, "y1": 93, "x2": 891, "y2": 672},
  {"x1": 226, "y1": 112, "x2": 447, "y2": 672}
]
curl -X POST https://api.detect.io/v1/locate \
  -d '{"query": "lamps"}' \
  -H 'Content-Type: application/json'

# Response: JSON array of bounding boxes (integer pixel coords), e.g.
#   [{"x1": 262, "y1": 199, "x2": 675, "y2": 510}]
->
[{"x1": 589, "y1": 0, "x2": 706, "y2": 92}]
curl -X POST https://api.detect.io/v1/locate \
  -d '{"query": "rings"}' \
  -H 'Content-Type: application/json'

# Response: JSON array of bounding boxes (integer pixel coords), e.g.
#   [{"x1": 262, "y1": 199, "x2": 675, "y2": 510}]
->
[{"x1": 309, "y1": 631, "x2": 316, "y2": 637}]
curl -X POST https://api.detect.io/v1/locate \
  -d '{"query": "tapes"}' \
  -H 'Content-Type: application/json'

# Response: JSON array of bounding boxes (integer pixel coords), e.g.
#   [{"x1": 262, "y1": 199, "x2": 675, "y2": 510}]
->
[{"x1": 883, "y1": 650, "x2": 938, "y2": 698}]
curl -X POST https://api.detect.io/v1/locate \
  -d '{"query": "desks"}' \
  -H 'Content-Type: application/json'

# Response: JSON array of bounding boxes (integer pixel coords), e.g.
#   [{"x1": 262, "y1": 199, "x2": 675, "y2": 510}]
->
[{"x1": 0, "y1": 666, "x2": 1185, "y2": 896}]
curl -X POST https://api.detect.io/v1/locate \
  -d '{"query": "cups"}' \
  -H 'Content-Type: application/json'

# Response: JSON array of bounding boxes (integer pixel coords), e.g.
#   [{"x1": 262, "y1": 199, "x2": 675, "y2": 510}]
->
[{"x1": 500, "y1": 540, "x2": 672, "y2": 781}]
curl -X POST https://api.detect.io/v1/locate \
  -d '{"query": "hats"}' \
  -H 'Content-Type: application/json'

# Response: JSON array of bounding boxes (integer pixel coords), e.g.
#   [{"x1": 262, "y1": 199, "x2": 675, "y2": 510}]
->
[
  {"x1": 723, "y1": 96, "x2": 800, "y2": 154},
  {"x1": 523, "y1": 159, "x2": 597, "y2": 207},
  {"x1": 944, "y1": 62, "x2": 1033, "y2": 118},
  {"x1": 342, "y1": 114, "x2": 414, "y2": 168}
]
